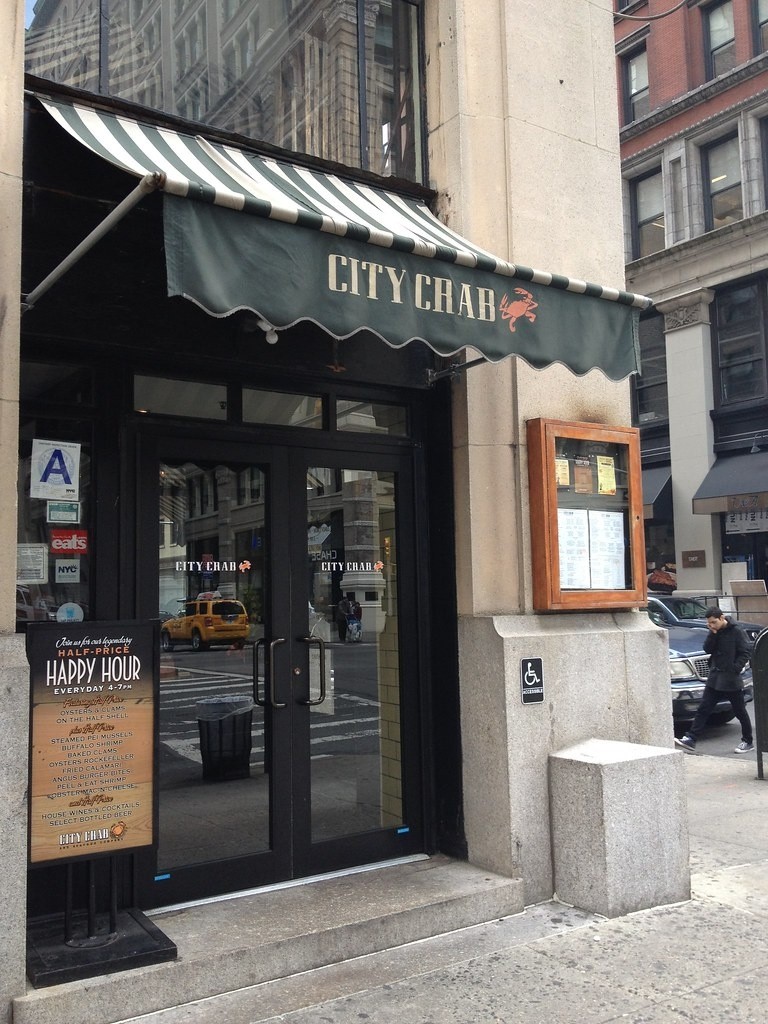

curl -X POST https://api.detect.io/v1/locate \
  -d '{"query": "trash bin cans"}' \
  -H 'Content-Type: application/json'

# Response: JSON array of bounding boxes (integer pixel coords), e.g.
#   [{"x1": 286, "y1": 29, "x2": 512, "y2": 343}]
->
[{"x1": 195, "y1": 696, "x2": 255, "y2": 782}]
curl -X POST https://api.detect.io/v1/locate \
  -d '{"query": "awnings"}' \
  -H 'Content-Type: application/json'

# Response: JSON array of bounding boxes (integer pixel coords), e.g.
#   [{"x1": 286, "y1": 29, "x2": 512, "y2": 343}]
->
[
  {"x1": 692, "y1": 450, "x2": 768, "y2": 514},
  {"x1": 20, "y1": 72, "x2": 650, "y2": 382},
  {"x1": 642, "y1": 462, "x2": 673, "y2": 521}
]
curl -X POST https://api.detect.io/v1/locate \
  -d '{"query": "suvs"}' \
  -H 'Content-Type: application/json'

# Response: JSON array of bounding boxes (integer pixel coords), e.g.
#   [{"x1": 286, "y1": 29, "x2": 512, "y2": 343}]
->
[
  {"x1": 642, "y1": 608, "x2": 754, "y2": 733},
  {"x1": 160, "y1": 590, "x2": 251, "y2": 650}
]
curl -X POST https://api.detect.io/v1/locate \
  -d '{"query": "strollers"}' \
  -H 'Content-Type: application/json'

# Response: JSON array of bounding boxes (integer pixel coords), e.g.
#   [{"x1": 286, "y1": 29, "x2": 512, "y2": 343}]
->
[{"x1": 346, "y1": 614, "x2": 363, "y2": 643}]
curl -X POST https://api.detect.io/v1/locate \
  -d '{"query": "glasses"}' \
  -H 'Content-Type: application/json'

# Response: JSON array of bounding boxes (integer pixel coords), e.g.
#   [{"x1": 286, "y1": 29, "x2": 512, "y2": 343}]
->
[{"x1": 345, "y1": 600, "x2": 348, "y2": 601}]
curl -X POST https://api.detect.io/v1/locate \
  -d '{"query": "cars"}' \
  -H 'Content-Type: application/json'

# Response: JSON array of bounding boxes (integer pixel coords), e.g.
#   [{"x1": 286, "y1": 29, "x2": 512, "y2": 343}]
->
[
  {"x1": 47, "y1": 606, "x2": 59, "y2": 622},
  {"x1": 646, "y1": 596, "x2": 766, "y2": 645},
  {"x1": 16, "y1": 584, "x2": 51, "y2": 622}
]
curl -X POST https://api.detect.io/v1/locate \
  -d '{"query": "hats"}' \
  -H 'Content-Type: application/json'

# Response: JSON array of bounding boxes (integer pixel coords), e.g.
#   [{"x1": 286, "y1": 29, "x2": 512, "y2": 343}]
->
[{"x1": 355, "y1": 602, "x2": 360, "y2": 605}]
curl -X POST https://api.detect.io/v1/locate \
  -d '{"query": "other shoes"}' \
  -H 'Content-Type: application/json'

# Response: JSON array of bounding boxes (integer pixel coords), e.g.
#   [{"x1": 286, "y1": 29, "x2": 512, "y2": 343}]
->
[{"x1": 341, "y1": 640, "x2": 344, "y2": 644}]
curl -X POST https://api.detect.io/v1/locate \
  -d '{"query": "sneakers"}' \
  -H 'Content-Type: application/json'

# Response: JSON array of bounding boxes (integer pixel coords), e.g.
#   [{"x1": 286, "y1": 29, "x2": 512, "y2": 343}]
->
[
  {"x1": 675, "y1": 736, "x2": 695, "y2": 751},
  {"x1": 734, "y1": 741, "x2": 754, "y2": 753}
]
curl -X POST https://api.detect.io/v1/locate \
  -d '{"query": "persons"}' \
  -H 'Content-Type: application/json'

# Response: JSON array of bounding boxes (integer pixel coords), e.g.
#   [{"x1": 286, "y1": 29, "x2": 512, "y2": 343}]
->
[
  {"x1": 348, "y1": 601, "x2": 362, "y2": 621},
  {"x1": 335, "y1": 596, "x2": 354, "y2": 642},
  {"x1": 674, "y1": 607, "x2": 755, "y2": 754}
]
